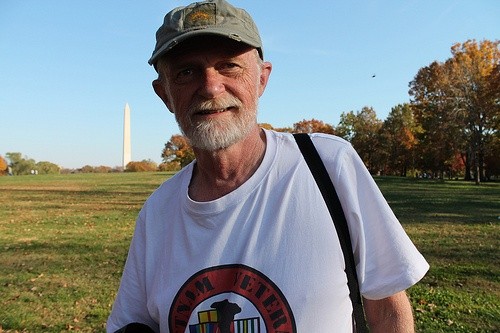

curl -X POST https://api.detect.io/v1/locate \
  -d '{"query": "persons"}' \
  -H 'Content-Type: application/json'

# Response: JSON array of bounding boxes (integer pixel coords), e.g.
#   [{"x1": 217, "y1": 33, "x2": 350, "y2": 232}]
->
[{"x1": 99, "y1": 2, "x2": 429, "y2": 333}]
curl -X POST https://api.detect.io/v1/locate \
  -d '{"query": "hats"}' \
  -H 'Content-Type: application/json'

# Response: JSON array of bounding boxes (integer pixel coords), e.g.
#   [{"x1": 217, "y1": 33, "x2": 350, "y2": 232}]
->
[{"x1": 147, "y1": 0, "x2": 264, "y2": 66}]
